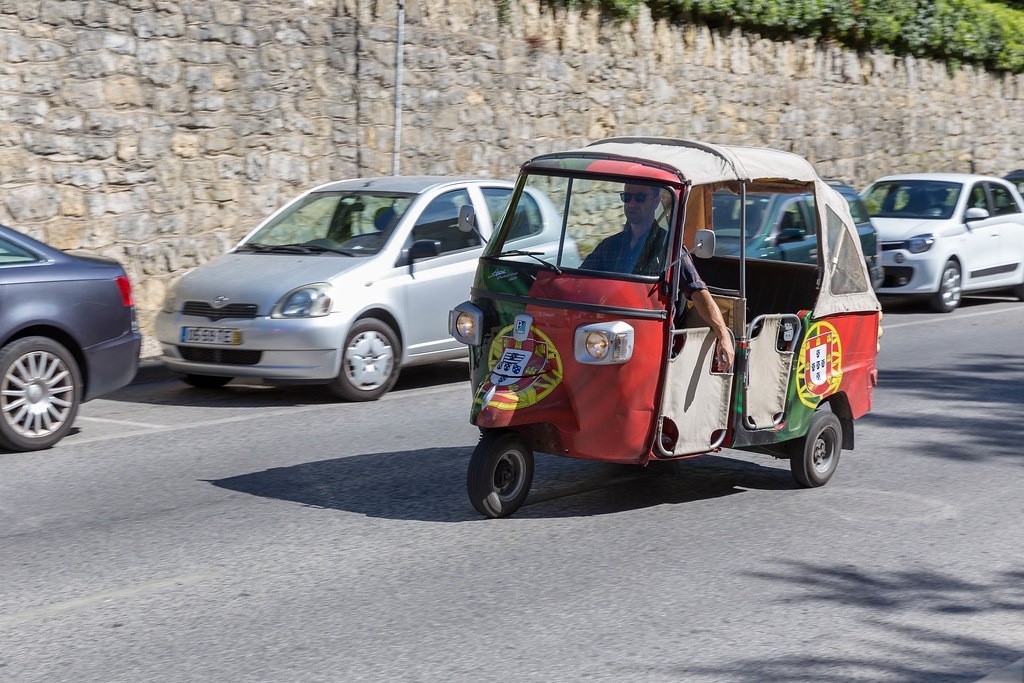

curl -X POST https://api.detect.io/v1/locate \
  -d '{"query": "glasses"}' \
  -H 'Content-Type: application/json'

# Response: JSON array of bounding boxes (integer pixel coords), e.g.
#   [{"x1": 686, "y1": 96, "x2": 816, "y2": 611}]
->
[{"x1": 620, "y1": 192, "x2": 654, "y2": 203}]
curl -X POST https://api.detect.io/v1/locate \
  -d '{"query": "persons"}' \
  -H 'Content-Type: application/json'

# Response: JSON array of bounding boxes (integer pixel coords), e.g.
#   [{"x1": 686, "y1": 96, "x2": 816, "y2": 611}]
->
[{"x1": 578, "y1": 181, "x2": 735, "y2": 375}]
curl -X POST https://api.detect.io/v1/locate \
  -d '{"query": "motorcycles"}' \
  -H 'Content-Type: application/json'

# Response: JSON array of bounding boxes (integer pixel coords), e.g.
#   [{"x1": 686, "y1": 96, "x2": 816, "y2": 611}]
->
[{"x1": 448, "y1": 136, "x2": 887, "y2": 520}]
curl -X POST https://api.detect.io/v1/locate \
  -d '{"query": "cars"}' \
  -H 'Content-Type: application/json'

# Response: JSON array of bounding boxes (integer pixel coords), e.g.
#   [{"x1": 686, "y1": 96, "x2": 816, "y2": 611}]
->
[
  {"x1": 0, "y1": 223, "x2": 144, "y2": 453},
  {"x1": 977, "y1": 169, "x2": 1024, "y2": 212},
  {"x1": 152, "y1": 175, "x2": 585, "y2": 402},
  {"x1": 847, "y1": 172, "x2": 1024, "y2": 313}
]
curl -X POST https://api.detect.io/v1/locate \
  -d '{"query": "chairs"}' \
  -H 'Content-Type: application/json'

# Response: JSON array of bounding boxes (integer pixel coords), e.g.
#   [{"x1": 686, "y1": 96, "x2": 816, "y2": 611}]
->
[
  {"x1": 416, "y1": 203, "x2": 467, "y2": 252},
  {"x1": 901, "y1": 190, "x2": 929, "y2": 214},
  {"x1": 780, "y1": 211, "x2": 795, "y2": 230},
  {"x1": 712, "y1": 206, "x2": 732, "y2": 229},
  {"x1": 374, "y1": 207, "x2": 395, "y2": 230},
  {"x1": 504, "y1": 205, "x2": 529, "y2": 241},
  {"x1": 739, "y1": 205, "x2": 761, "y2": 232}
]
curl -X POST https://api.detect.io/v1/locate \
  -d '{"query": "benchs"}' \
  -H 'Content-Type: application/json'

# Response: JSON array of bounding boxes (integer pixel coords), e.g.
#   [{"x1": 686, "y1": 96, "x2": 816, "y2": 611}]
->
[{"x1": 688, "y1": 253, "x2": 822, "y2": 350}]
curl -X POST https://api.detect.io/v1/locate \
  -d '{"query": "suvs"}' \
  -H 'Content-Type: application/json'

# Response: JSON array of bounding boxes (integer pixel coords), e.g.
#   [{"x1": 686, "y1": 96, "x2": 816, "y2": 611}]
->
[{"x1": 710, "y1": 177, "x2": 886, "y2": 295}]
